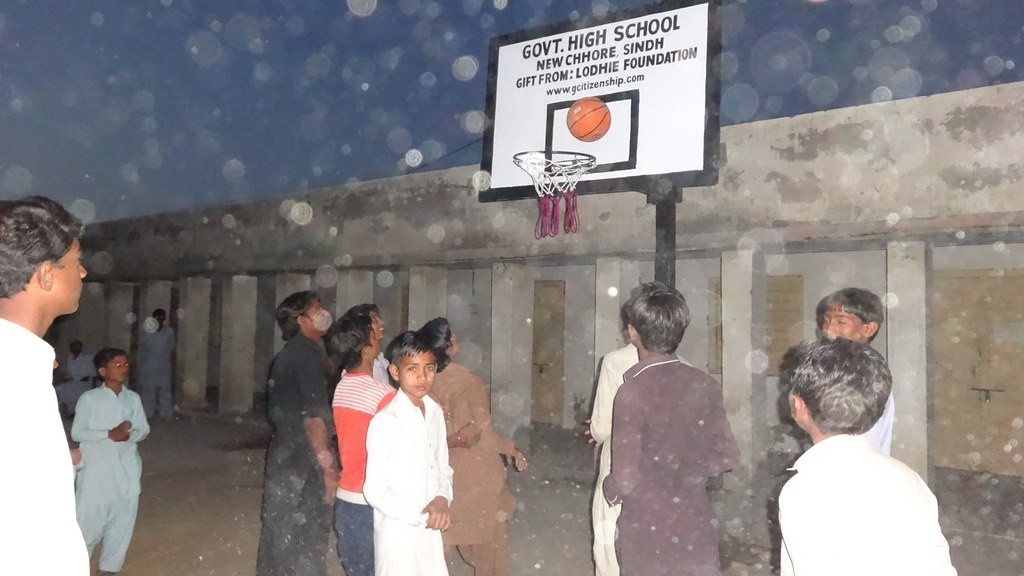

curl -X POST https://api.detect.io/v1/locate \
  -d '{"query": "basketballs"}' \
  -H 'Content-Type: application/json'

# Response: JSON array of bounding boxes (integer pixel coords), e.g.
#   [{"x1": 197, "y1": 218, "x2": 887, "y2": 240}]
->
[{"x1": 566, "y1": 96, "x2": 612, "y2": 143}]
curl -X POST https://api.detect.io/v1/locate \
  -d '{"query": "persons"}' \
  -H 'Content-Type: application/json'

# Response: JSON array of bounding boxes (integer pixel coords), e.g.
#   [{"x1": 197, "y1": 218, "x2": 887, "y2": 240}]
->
[
  {"x1": 71, "y1": 348, "x2": 149, "y2": 576},
  {"x1": 583, "y1": 282, "x2": 949, "y2": 576},
  {"x1": 255, "y1": 291, "x2": 529, "y2": 576},
  {"x1": 141, "y1": 309, "x2": 175, "y2": 420},
  {"x1": 1, "y1": 196, "x2": 92, "y2": 576}
]
